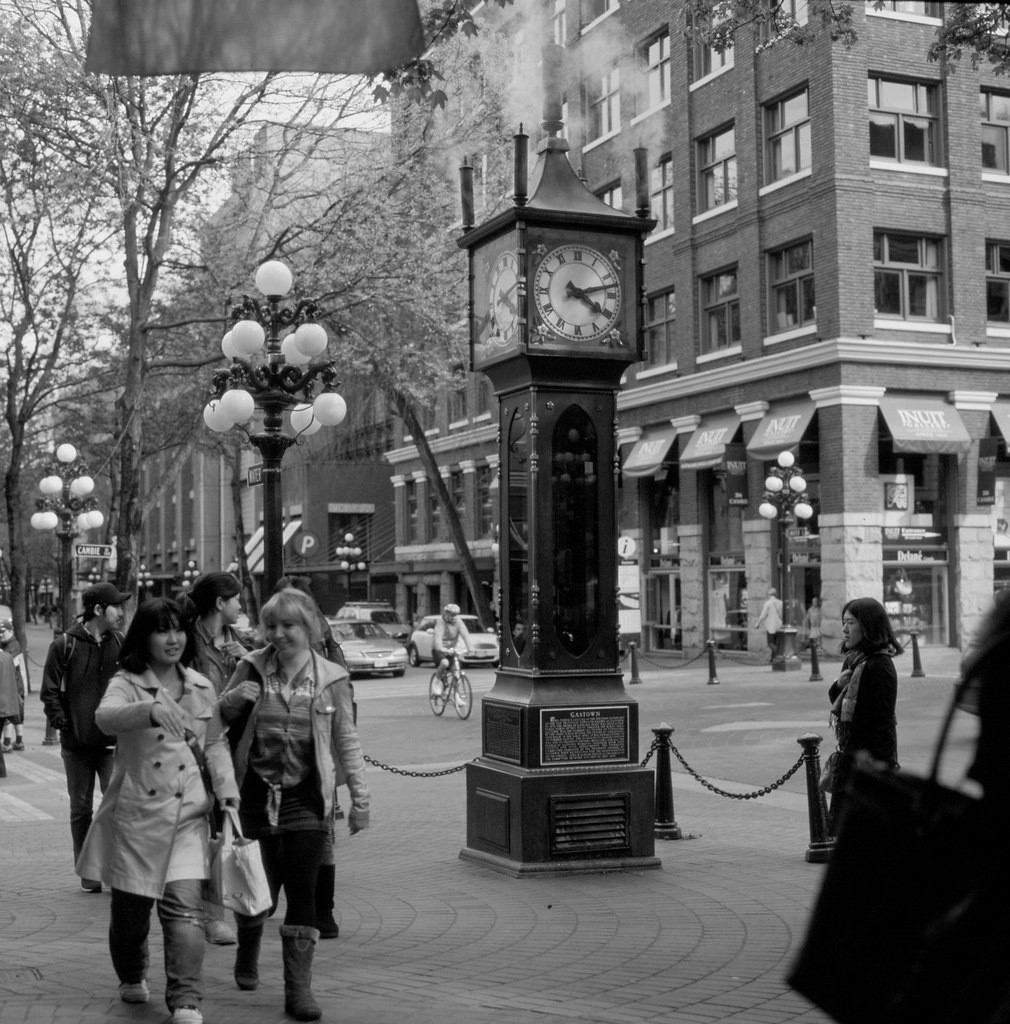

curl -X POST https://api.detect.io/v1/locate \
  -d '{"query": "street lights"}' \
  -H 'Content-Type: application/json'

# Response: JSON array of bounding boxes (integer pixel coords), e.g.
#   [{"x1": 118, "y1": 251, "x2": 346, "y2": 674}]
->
[
  {"x1": 202, "y1": 260, "x2": 348, "y2": 611},
  {"x1": 29, "y1": 443, "x2": 105, "y2": 634},
  {"x1": 336, "y1": 533, "x2": 367, "y2": 602},
  {"x1": 757, "y1": 451, "x2": 814, "y2": 670}
]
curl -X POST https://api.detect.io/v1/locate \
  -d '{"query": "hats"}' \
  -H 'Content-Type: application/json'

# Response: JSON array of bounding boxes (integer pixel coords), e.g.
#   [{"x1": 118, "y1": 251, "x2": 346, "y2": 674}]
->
[
  {"x1": 81, "y1": 583, "x2": 131, "y2": 607},
  {"x1": 0, "y1": 619, "x2": 14, "y2": 630}
]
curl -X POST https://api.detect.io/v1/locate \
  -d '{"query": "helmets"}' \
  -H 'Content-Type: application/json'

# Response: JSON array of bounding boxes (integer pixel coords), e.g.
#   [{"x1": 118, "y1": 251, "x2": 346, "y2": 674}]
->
[{"x1": 444, "y1": 603, "x2": 461, "y2": 621}]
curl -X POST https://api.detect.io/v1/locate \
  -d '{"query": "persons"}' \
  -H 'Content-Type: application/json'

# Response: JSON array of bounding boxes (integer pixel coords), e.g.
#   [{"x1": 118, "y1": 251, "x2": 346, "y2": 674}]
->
[
  {"x1": 218, "y1": 588, "x2": 370, "y2": 1021},
  {"x1": 74, "y1": 597, "x2": 241, "y2": 1024},
  {"x1": 806, "y1": 597, "x2": 821, "y2": 638},
  {"x1": 754, "y1": 588, "x2": 783, "y2": 662},
  {"x1": 176, "y1": 571, "x2": 256, "y2": 945},
  {"x1": 37, "y1": 578, "x2": 59, "y2": 615},
  {"x1": 431, "y1": 604, "x2": 473, "y2": 707},
  {"x1": 256, "y1": 575, "x2": 354, "y2": 938},
  {"x1": 40, "y1": 582, "x2": 132, "y2": 893},
  {"x1": 828, "y1": 598, "x2": 897, "y2": 769},
  {"x1": 968, "y1": 589, "x2": 1010, "y2": 890},
  {"x1": 0, "y1": 605, "x2": 30, "y2": 777}
]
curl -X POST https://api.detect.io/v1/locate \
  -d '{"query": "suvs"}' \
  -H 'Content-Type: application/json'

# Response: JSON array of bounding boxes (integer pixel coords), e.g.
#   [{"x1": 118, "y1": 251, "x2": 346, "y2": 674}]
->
[{"x1": 333, "y1": 600, "x2": 414, "y2": 646}]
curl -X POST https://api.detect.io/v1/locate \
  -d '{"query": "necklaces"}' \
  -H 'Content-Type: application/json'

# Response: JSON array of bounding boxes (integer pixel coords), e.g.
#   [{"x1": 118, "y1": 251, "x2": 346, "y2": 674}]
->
[{"x1": 163, "y1": 688, "x2": 169, "y2": 694}]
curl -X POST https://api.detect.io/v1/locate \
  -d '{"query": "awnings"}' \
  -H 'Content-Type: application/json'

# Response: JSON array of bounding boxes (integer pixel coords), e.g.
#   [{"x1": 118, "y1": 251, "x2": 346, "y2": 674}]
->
[
  {"x1": 746, "y1": 396, "x2": 816, "y2": 461},
  {"x1": 621, "y1": 423, "x2": 676, "y2": 481},
  {"x1": 878, "y1": 398, "x2": 972, "y2": 455},
  {"x1": 991, "y1": 395, "x2": 1010, "y2": 454},
  {"x1": 679, "y1": 411, "x2": 741, "y2": 470}
]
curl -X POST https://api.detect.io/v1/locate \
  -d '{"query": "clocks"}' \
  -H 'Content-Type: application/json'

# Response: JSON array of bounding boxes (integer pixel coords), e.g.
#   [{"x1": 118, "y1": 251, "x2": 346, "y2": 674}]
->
[
  {"x1": 519, "y1": 207, "x2": 656, "y2": 357},
  {"x1": 470, "y1": 217, "x2": 529, "y2": 369}
]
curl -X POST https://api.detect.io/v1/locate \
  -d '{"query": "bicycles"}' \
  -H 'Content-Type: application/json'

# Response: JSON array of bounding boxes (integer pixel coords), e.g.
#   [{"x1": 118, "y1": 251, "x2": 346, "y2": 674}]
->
[{"x1": 428, "y1": 648, "x2": 474, "y2": 721}]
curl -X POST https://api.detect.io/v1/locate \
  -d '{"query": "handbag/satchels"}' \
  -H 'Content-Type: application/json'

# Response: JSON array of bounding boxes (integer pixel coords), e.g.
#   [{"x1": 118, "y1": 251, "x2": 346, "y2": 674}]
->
[
  {"x1": 818, "y1": 751, "x2": 843, "y2": 793},
  {"x1": 198, "y1": 805, "x2": 273, "y2": 917}
]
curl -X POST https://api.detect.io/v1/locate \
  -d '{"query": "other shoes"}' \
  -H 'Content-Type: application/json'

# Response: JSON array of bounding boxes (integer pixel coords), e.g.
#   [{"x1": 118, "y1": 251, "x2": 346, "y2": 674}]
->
[
  {"x1": 79, "y1": 877, "x2": 102, "y2": 893},
  {"x1": 13, "y1": 742, "x2": 25, "y2": 751},
  {"x1": 451, "y1": 694, "x2": 465, "y2": 707},
  {"x1": 0, "y1": 763, "x2": 7, "y2": 777},
  {"x1": 432, "y1": 676, "x2": 442, "y2": 696},
  {"x1": 172, "y1": 1004, "x2": 203, "y2": 1024},
  {"x1": 117, "y1": 978, "x2": 150, "y2": 1003},
  {"x1": 1, "y1": 742, "x2": 14, "y2": 753}
]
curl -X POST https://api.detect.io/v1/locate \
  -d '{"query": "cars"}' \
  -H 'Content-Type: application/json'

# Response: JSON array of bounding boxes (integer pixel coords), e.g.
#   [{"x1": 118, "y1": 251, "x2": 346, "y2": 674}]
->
[
  {"x1": 405, "y1": 614, "x2": 500, "y2": 671},
  {"x1": 325, "y1": 616, "x2": 410, "y2": 680},
  {"x1": 229, "y1": 613, "x2": 264, "y2": 650}
]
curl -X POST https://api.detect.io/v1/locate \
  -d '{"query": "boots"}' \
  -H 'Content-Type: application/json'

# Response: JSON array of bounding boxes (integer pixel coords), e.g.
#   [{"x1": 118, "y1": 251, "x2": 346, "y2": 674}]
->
[
  {"x1": 233, "y1": 909, "x2": 268, "y2": 990},
  {"x1": 313, "y1": 864, "x2": 340, "y2": 938},
  {"x1": 278, "y1": 923, "x2": 321, "y2": 1021},
  {"x1": 200, "y1": 882, "x2": 234, "y2": 943}
]
